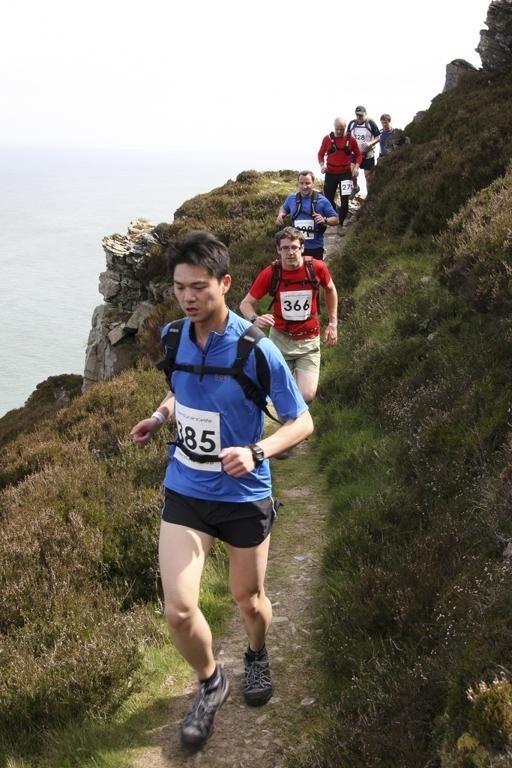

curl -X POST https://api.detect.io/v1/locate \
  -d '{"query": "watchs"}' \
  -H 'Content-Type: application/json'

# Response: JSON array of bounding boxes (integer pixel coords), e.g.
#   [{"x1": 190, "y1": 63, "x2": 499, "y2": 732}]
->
[{"x1": 245, "y1": 444, "x2": 265, "y2": 469}]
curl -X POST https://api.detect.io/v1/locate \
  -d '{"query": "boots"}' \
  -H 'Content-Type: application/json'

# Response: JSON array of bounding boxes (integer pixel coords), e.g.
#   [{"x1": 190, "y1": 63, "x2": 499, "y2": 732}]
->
[{"x1": 337, "y1": 225, "x2": 346, "y2": 236}]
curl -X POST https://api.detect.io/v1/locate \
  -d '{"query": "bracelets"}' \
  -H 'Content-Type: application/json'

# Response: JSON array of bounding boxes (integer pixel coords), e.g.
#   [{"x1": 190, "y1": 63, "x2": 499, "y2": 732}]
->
[
  {"x1": 250, "y1": 314, "x2": 258, "y2": 324},
  {"x1": 151, "y1": 412, "x2": 166, "y2": 424},
  {"x1": 328, "y1": 322, "x2": 338, "y2": 327}
]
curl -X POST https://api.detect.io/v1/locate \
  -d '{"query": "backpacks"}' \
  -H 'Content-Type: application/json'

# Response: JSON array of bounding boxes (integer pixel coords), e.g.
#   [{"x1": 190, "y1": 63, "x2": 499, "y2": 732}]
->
[{"x1": 291, "y1": 189, "x2": 327, "y2": 233}]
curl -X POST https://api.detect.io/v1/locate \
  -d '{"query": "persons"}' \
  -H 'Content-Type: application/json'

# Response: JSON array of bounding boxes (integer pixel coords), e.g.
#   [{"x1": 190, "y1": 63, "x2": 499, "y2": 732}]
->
[
  {"x1": 129, "y1": 230, "x2": 315, "y2": 751},
  {"x1": 238, "y1": 226, "x2": 339, "y2": 405},
  {"x1": 317, "y1": 105, "x2": 399, "y2": 231},
  {"x1": 277, "y1": 170, "x2": 340, "y2": 260}
]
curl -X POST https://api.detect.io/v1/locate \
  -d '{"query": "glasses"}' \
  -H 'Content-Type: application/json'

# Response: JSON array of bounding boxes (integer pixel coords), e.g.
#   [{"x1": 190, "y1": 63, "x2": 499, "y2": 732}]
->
[{"x1": 279, "y1": 245, "x2": 301, "y2": 253}]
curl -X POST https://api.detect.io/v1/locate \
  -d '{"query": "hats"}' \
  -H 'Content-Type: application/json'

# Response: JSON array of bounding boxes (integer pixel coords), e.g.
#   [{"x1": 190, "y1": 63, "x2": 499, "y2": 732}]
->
[{"x1": 356, "y1": 106, "x2": 366, "y2": 115}]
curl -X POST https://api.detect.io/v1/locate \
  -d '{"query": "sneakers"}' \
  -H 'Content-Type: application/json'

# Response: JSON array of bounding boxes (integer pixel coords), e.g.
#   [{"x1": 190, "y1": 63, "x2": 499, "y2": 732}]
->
[
  {"x1": 181, "y1": 673, "x2": 231, "y2": 746},
  {"x1": 348, "y1": 187, "x2": 360, "y2": 200},
  {"x1": 242, "y1": 652, "x2": 273, "y2": 707}
]
